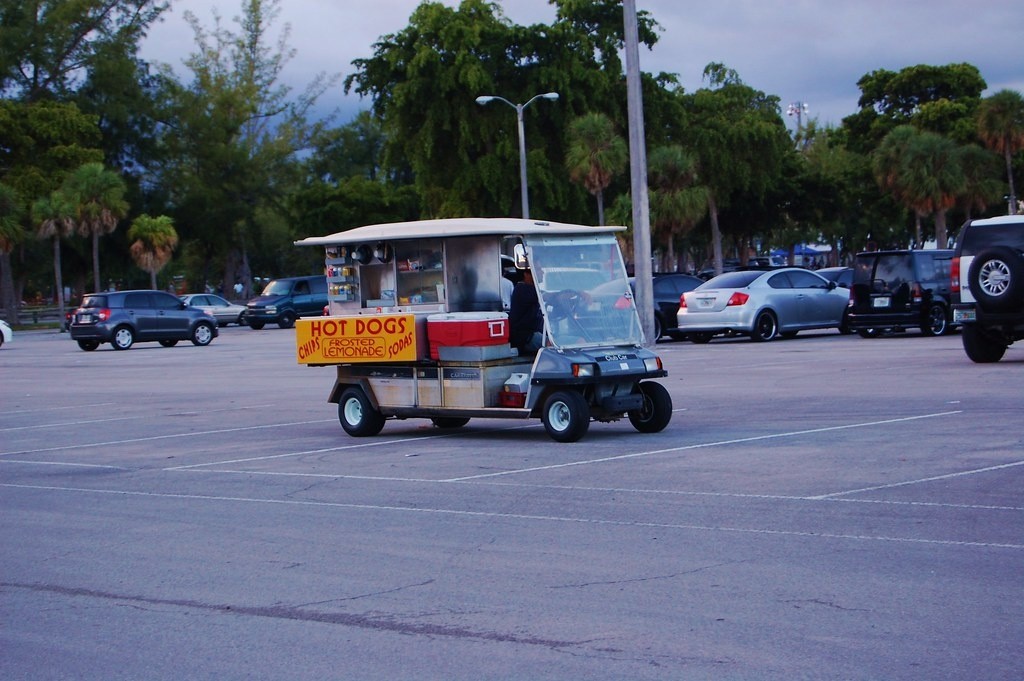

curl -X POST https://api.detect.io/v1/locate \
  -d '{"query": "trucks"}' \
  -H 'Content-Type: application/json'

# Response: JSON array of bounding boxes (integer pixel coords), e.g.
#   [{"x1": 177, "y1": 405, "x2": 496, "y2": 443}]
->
[{"x1": 294, "y1": 217, "x2": 672, "y2": 443}]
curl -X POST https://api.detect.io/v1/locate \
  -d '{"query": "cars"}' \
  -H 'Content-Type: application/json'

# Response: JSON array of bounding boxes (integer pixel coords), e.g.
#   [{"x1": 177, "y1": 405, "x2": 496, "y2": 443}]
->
[
  {"x1": 697, "y1": 258, "x2": 759, "y2": 280},
  {"x1": 794, "y1": 266, "x2": 854, "y2": 289},
  {"x1": 176, "y1": 294, "x2": 246, "y2": 326},
  {"x1": 677, "y1": 267, "x2": 850, "y2": 342},
  {"x1": 746, "y1": 256, "x2": 790, "y2": 266},
  {"x1": 590, "y1": 274, "x2": 706, "y2": 339}
]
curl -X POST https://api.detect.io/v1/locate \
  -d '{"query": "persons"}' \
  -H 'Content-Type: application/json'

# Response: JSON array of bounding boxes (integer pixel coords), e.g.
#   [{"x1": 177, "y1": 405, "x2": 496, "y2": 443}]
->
[
  {"x1": 803, "y1": 257, "x2": 816, "y2": 271},
  {"x1": 103, "y1": 276, "x2": 267, "y2": 299},
  {"x1": 509, "y1": 255, "x2": 591, "y2": 355}
]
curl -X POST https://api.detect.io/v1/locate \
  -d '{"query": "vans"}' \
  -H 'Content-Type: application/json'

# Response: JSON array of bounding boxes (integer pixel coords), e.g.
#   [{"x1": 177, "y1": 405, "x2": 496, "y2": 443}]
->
[
  {"x1": 69, "y1": 289, "x2": 218, "y2": 350},
  {"x1": 538, "y1": 268, "x2": 607, "y2": 294}
]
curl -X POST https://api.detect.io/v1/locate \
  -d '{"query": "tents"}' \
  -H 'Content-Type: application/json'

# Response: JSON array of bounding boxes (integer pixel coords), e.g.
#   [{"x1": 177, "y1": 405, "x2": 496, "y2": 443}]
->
[{"x1": 769, "y1": 243, "x2": 834, "y2": 255}]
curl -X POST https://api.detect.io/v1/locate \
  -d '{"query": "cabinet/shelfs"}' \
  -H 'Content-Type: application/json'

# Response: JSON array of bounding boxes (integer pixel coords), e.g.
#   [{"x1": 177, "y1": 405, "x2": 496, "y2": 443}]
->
[{"x1": 323, "y1": 248, "x2": 356, "y2": 302}]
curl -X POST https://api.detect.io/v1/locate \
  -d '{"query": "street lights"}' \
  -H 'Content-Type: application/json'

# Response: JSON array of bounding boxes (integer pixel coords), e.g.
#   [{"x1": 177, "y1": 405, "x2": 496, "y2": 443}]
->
[{"x1": 476, "y1": 92, "x2": 559, "y2": 219}]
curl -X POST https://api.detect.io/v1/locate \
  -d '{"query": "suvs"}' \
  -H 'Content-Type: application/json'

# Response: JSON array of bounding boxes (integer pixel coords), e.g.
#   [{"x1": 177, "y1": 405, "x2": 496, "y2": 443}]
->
[
  {"x1": 848, "y1": 249, "x2": 955, "y2": 338},
  {"x1": 950, "y1": 214, "x2": 1024, "y2": 364},
  {"x1": 242, "y1": 275, "x2": 329, "y2": 330}
]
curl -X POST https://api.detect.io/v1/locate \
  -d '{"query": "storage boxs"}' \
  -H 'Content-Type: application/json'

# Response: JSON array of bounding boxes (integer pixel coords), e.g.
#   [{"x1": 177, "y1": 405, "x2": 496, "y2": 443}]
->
[{"x1": 427, "y1": 312, "x2": 508, "y2": 361}]
